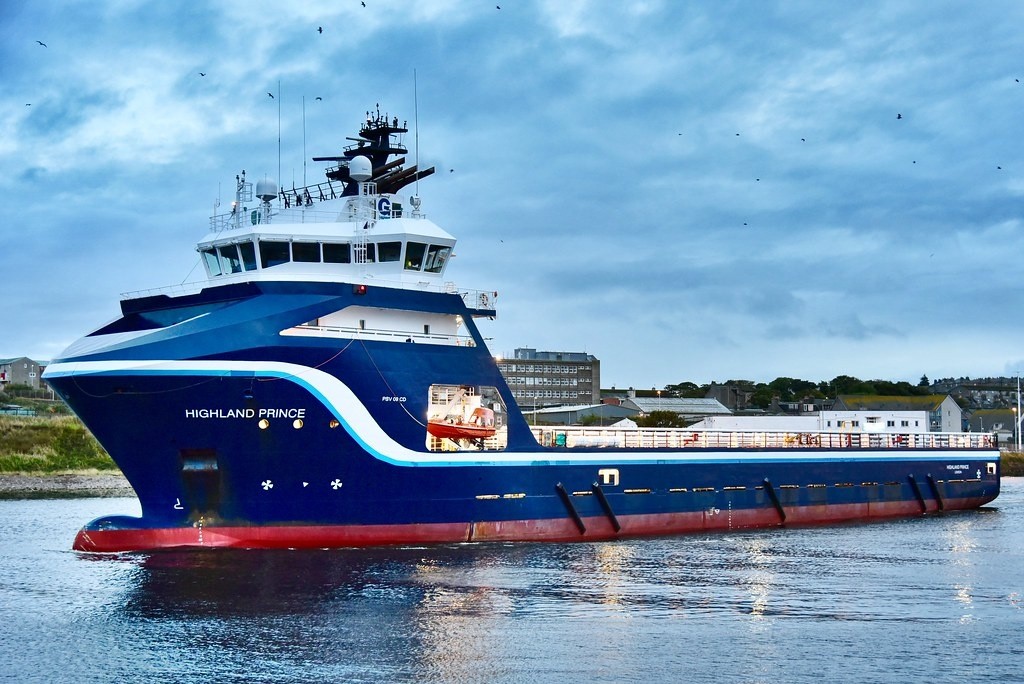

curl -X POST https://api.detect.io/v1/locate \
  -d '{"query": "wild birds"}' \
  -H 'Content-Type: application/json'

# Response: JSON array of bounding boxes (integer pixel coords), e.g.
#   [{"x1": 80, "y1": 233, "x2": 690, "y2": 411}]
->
[{"x1": 0, "y1": 0, "x2": 1024, "y2": 243}]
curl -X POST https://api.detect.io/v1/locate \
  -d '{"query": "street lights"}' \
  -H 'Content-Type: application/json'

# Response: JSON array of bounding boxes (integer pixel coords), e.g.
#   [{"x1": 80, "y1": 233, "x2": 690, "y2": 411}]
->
[
  {"x1": 1012, "y1": 407, "x2": 1017, "y2": 453},
  {"x1": 657, "y1": 390, "x2": 661, "y2": 411},
  {"x1": 533, "y1": 397, "x2": 538, "y2": 426}
]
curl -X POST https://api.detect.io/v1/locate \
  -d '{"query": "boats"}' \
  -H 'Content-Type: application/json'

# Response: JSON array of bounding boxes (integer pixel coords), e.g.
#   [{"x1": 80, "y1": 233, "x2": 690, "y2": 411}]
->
[{"x1": 426, "y1": 406, "x2": 497, "y2": 440}]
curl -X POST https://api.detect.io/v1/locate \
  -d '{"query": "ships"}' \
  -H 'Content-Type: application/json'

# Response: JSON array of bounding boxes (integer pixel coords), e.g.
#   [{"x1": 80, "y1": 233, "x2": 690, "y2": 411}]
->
[{"x1": 39, "y1": 66, "x2": 1001, "y2": 556}]
